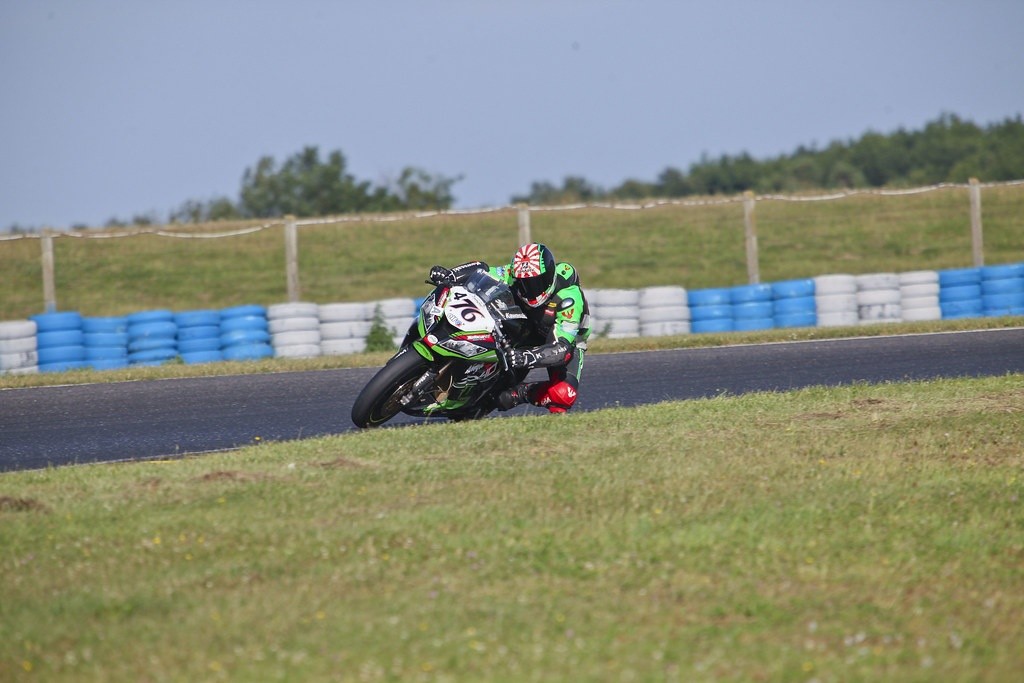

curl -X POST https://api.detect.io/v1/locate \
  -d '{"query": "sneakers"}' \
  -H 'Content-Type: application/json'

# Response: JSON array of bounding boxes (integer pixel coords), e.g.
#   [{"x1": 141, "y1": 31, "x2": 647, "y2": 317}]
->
[{"x1": 499, "y1": 381, "x2": 533, "y2": 412}]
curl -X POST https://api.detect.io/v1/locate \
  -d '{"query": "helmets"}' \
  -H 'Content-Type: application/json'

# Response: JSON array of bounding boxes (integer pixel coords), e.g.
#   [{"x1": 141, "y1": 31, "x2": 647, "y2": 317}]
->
[{"x1": 510, "y1": 243, "x2": 557, "y2": 307}]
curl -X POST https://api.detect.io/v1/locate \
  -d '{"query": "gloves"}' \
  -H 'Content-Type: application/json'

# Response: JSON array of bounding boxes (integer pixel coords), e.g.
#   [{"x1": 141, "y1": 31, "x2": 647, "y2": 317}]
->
[
  {"x1": 428, "y1": 265, "x2": 456, "y2": 284},
  {"x1": 504, "y1": 345, "x2": 536, "y2": 368}
]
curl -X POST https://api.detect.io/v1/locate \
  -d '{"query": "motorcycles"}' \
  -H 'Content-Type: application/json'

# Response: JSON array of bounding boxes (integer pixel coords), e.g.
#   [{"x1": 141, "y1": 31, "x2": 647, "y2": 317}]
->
[{"x1": 351, "y1": 268, "x2": 530, "y2": 428}]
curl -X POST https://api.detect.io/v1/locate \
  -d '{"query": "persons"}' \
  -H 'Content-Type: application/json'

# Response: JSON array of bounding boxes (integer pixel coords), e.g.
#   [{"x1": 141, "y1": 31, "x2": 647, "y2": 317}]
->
[{"x1": 429, "y1": 245, "x2": 590, "y2": 410}]
